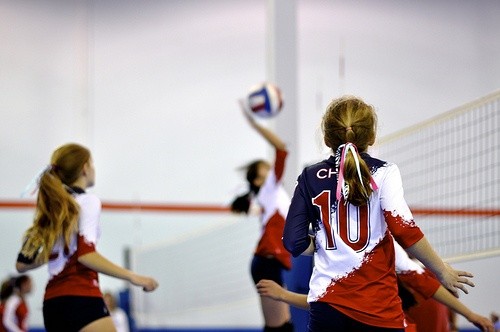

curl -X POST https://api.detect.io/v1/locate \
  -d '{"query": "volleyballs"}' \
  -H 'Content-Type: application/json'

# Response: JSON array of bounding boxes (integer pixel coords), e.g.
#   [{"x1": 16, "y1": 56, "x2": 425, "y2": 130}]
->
[{"x1": 249, "y1": 82, "x2": 284, "y2": 119}]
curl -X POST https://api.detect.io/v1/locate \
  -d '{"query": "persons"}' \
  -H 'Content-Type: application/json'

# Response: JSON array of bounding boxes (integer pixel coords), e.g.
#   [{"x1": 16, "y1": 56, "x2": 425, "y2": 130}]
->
[
  {"x1": 104, "y1": 292, "x2": 129, "y2": 332},
  {"x1": 257, "y1": 97, "x2": 496, "y2": 332},
  {"x1": 0, "y1": 275, "x2": 32, "y2": 332},
  {"x1": 16, "y1": 144, "x2": 159, "y2": 332},
  {"x1": 230, "y1": 98, "x2": 295, "y2": 332}
]
